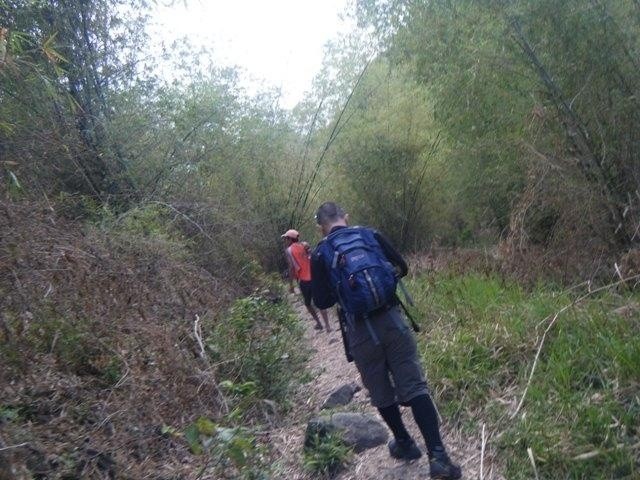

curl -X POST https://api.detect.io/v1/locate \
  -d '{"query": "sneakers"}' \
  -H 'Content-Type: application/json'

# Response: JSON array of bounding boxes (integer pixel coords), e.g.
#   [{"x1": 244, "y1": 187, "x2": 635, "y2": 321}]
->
[
  {"x1": 430, "y1": 458, "x2": 461, "y2": 480},
  {"x1": 388, "y1": 439, "x2": 421, "y2": 459}
]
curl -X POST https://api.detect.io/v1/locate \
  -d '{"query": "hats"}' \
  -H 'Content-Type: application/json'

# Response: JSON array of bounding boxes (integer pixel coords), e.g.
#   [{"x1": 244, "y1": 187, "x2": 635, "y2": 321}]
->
[{"x1": 281, "y1": 229, "x2": 299, "y2": 239}]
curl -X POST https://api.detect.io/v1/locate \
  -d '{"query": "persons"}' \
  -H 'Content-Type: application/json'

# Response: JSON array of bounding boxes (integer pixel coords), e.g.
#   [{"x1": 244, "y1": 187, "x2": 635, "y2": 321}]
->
[
  {"x1": 310, "y1": 201, "x2": 463, "y2": 480},
  {"x1": 280, "y1": 229, "x2": 332, "y2": 333}
]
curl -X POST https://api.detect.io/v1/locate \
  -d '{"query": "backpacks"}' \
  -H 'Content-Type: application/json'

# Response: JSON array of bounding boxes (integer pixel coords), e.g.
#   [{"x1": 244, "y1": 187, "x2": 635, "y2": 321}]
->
[{"x1": 317, "y1": 225, "x2": 398, "y2": 312}]
